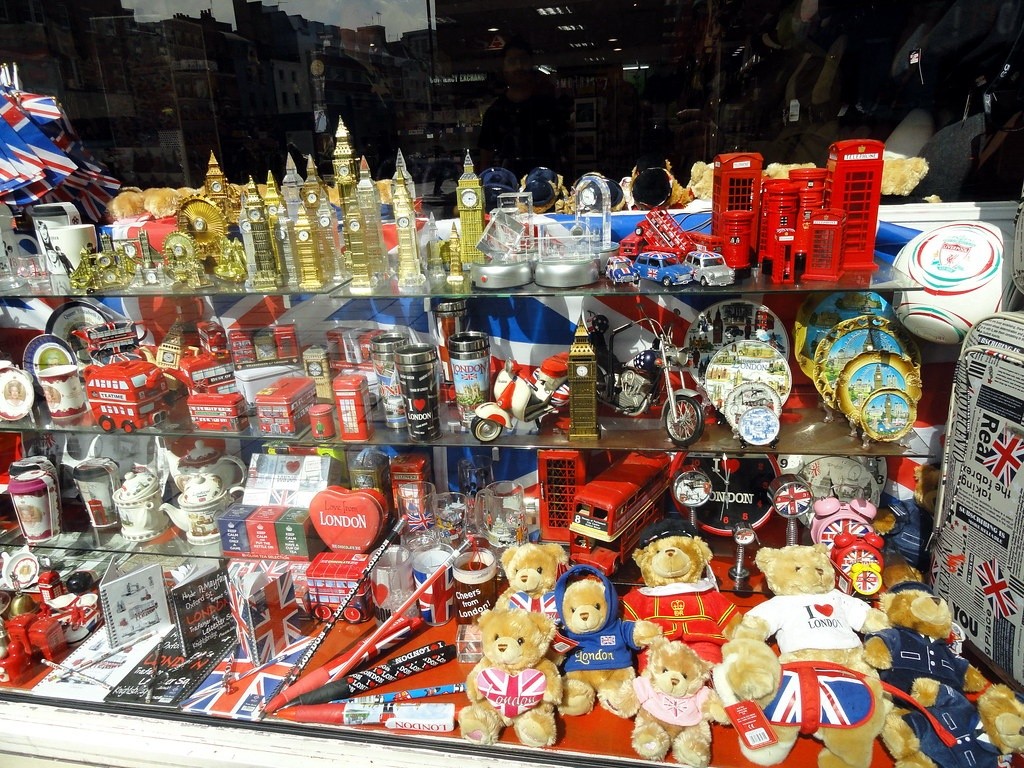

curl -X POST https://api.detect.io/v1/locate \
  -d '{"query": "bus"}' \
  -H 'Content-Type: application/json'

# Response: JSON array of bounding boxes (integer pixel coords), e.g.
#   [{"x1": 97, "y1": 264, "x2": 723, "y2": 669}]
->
[
  {"x1": 567, "y1": 452, "x2": 673, "y2": 579},
  {"x1": 620, "y1": 205, "x2": 723, "y2": 264},
  {"x1": 84, "y1": 362, "x2": 173, "y2": 433}
]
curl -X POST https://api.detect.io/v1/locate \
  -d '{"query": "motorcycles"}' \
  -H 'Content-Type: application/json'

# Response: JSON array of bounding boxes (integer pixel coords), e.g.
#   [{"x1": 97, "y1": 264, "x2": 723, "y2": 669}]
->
[{"x1": 596, "y1": 304, "x2": 705, "y2": 447}]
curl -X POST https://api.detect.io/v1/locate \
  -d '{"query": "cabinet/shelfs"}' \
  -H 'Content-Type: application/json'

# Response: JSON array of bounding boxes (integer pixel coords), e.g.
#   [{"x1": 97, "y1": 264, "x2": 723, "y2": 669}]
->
[{"x1": 0, "y1": 252, "x2": 1024, "y2": 768}]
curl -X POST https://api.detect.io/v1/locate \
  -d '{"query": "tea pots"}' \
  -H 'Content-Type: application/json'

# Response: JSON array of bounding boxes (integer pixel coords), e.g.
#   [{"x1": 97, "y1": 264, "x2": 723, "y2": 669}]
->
[
  {"x1": 176, "y1": 440, "x2": 247, "y2": 500},
  {"x1": 159, "y1": 472, "x2": 246, "y2": 546},
  {"x1": 112, "y1": 471, "x2": 165, "y2": 542}
]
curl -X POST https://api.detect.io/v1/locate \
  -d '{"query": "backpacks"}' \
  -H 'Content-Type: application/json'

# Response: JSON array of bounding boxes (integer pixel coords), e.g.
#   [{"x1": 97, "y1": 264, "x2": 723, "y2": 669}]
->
[{"x1": 924, "y1": 309, "x2": 1024, "y2": 698}]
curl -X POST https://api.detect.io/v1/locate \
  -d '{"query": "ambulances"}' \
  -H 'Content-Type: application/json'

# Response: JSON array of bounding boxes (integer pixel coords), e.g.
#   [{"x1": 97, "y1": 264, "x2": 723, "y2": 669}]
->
[
  {"x1": 683, "y1": 246, "x2": 736, "y2": 288},
  {"x1": 633, "y1": 252, "x2": 694, "y2": 286},
  {"x1": 604, "y1": 256, "x2": 640, "y2": 285}
]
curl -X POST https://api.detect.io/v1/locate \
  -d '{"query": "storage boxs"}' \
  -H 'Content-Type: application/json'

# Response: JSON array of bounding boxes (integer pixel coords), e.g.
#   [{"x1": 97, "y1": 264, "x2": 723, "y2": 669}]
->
[
  {"x1": 275, "y1": 506, "x2": 318, "y2": 557},
  {"x1": 306, "y1": 553, "x2": 374, "y2": 623},
  {"x1": 217, "y1": 504, "x2": 255, "y2": 552},
  {"x1": 246, "y1": 506, "x2": 289, "y2": 555},
  {"x1": 234, "y1": 363, "x2": 305, "y2": 408}
]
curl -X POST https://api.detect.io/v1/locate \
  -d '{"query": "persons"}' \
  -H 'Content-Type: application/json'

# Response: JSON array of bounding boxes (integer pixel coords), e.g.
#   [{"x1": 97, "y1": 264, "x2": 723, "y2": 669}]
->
[{"x1": 479, "y1": 40, "x2": 574, "y2": 184}]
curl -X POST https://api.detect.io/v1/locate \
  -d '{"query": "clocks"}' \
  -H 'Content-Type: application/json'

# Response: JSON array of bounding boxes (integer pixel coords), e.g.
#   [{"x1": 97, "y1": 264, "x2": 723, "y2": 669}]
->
[
  {"x1": 268, "y1": 205, "x2": 277, "y2": 215},
  {"x1": 339, "y1": 165, "x2": 349, "y2": 177},
  {"x1": 774, "y1": 453, "x2": 888, "y2": 495},
  {"x1": 124, "y1": 244, "x2": 136, "y2": 258},
  {"x1": 275, "y1": 229, "x2": 286, "y2": 240},
  {"x1": 349, "y1": 221, "x2": 361, "y2": 233},
  {"x1": 574, "y1": 366, "x2": 588, "y2": 378},
  {"x1": 298, "y1": 230, "x2": 322, "y2": 376},
  {"x1": 212, "y1": 182, "x2": 221, "y2": 191},
  {"x1": 196, "y1": 221, "x2": 203, "y2": 230},
  {"x1": 320, "y1": 216, "x2": 331, "y2": 228},
  {"x1": 398, "y1": 218, "x2": 409, "y2": 228},
  {"x1": 670, "y1": 452, "x2": 782, "y2": 547},
  {"x1": 241, "y1": 221, "x2": 251, "y2": 232},
  {"x1": 99, "y1": 257, "x2": 110, "y2": 266},
  {"x1": 104, "y1": 271, "x2": 115, "y2": 283},
  {"x1": 810, "y1": 495, "x2": 884, "y2": 596},
  {"x1": 582, "y1": 189, "x2": 594, "y2": 204},
  {"x1": 462, "y1": 192, "x2": 478, "y2": 208},
  {"x1": 146, "y1": 271, "x2": 175, "y2": 364},
  {"x1": 308, "y1": 194, "x2": 317, "y2": 203},
  {"x1": 682, "y1": 289, "x2": 922, "y2": 451},
  {"x1": 249, "y1": 210, "x2": 260, "y2": 221}
]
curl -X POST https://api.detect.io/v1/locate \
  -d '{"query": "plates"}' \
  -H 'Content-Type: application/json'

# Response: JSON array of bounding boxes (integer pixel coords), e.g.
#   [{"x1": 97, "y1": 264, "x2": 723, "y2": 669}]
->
[
  {"x1": 860, "y1": 387, "x2": 917, "y2": 440},
  {"x1": 45, "y1": 300, "x2": 109, "y2": 369},
  {"x1": 813, "y1": 315, "x2": 921, "y2": 412},
  {"x1": 725, "y1": 382, "x2": 783, "y2": 426},
  {"x1": 792, "y1": 292, "x2": 896, "y2": 380},
  {"x1": 0, "y1": 368, "x2": 34, "y2": 422},
  {"x1": 739, "y1": 406, "x2": 779, "y2": 445},
  {"x1": 685, "y1": 298, "x2": 790, "y2": 390},
  {"x1": 705, "y1": 340, "x2": 792, "y2": 414},
  {"x1": 796, "y1": 458, "x2": 880, "y2": 528},
  {"x1": 23, "y1": 334, "x2": 77, "y2": 397},
  {"x1": 835, "y1": 349, "x2": 922, "y2": 427}
]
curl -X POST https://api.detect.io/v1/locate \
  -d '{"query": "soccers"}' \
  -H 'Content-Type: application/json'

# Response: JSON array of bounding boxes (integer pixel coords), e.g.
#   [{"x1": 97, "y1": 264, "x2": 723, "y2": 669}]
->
[{"x1": 889, "y1": 218, "x2": 1021, "y2": 346}]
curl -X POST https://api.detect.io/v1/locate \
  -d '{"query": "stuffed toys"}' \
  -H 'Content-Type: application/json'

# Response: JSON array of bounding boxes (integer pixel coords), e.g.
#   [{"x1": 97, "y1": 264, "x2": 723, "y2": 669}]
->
[
  {"x1": 480, "y1": 151, "x2": 944, "y2": 216},
  {"x1": 456, "y1": 462, "x2": 1024, "y2": 768}
]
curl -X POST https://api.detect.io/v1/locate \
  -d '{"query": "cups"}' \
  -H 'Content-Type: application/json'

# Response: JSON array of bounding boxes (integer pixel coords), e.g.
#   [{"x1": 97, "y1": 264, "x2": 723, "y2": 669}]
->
[
  {"x1": 8, "y1": 455, "x2": 58, "y2": 478},
  {"x1": 452, "y1": 549, "x2": 497, "y2": 627},
  {"x1": 432, "y1": 491, "x2": 467, "y2": 552},
  {"x1": 394, "y1": 343, "x2": 443, "y2": 443},
  {"x1": 432, "y1": 298, "x2": 473, "y2": 384},
  {"x1": 370, "y1": 332, "x2": 409, "y2": 428},
  {"x1": 31, "y1": 203, "x2": 68, "y2": 274},
  {"x1": 369, "y1": 543, "x2": 413, "y2": 628},
  {"x1": 458, "y1": 454, "x2": 494, "y2": 534},
  {"x1": 48, "y1": 224, "x2": 98, "y2": 278},
  {"x1": 411, "y1": 543, "x2": 454, "y2": 627},
  {"x1": 448, "y1": 331, "x2": 491, "y2": 429},
  {"x1": 72, "y1": 457, "x2": 120, "y2": 526},
  {"x1": 7, "y1": 476, "x2": 52, "y2": 543},
  {"x1": 0, "y1": 231, "x2": 42, "y2": 292},
  {"x1": 19, "y1": 255, "x2": 44, "y2": 285},
  {"x1": 37, "y1": 366, "x2": 86, "y2": 418},
  {"x1": 397, "y1": 481, "x2": 440, "y2": 553},
  {"x1": 474, "y1": 481, "x2": 528, "y2": 580}
]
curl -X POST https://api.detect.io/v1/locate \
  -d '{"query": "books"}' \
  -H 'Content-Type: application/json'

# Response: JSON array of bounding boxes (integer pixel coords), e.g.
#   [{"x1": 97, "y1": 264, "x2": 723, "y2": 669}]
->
[{"x1": 31, "y1": 563, "x2": 328, "y2": 722}]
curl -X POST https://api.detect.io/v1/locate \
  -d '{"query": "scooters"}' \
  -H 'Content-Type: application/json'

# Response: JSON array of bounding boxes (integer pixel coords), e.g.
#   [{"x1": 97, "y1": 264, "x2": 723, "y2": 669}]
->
[{"x1": 470, "y1": 352, "x2": 571, "y2": 442}]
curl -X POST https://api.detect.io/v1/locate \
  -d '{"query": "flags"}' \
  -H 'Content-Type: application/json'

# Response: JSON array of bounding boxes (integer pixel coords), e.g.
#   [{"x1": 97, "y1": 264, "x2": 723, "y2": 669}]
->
[{"x1": 0, "y1": 63, "x2": 120, "y2": 223}]
